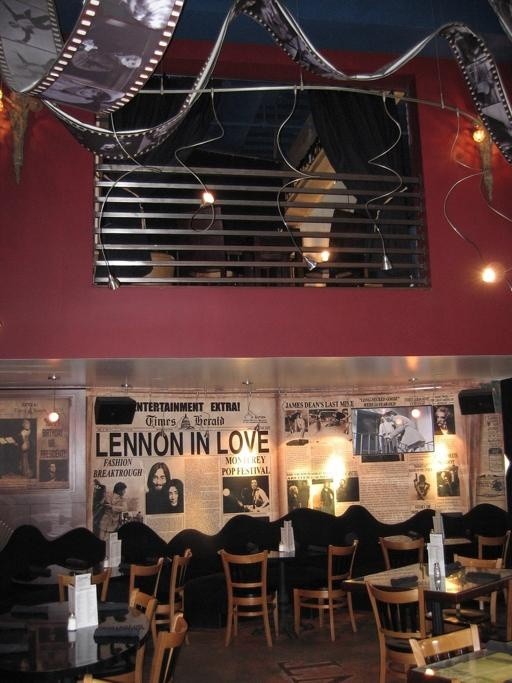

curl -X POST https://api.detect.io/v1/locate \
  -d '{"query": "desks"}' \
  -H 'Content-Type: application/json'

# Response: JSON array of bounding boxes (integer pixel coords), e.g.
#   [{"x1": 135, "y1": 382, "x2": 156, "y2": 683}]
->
[
  {"x1": 342, "y1": 561, "x2": 512, "y2": 637},
  {"x1": 406, "y1": 640, "x2": 512, "y2": 682},
  {"x1": 241, "y1": 544, "x2": 329, "y2": 642},
  {"x1": 1, "y1": 597, "x2": 145, "y2": 682}
]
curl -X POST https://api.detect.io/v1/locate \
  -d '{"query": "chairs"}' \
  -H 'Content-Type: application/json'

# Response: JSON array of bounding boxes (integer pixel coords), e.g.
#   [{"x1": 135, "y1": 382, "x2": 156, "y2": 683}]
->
[
  {"x1": 406, "y1": 622, "x2": 482, "y2": 668},
  {"x1": 364, "y1": 580, "x2": 433, "y2": 682},
  {"x1": 155, "y1": 548, "x2": 194, "y2": 646},
  {"x1": 218, "y1": 549, "x2": 281, "y2": 649},
  {"x1": 81, "y1": 612, "x2": 188, "y2": 683},
  {"x1": 126, "y1": 556, "x2": 165, "y2": 648},
  {"x1": 56, "y1": 569, "x2": 110, "y2": 600},
  {"x1": 476, "y1": 532, "x2": 511, "y2": 568},
  {"x1": 426, "y1": 552, "x2": 504, "y2": 628},
  {"x1": 378, "y1": 536, "x2": 425, "y2": 569},
  {"x1": 291, "y1": 538, "x2": 360, "y2": 641}
]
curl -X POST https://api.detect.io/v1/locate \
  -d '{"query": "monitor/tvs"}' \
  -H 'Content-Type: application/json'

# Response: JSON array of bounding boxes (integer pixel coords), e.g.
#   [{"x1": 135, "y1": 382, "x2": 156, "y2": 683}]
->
[{"x1": 351, "y1": 405, "x2": 434, "y2": 462}]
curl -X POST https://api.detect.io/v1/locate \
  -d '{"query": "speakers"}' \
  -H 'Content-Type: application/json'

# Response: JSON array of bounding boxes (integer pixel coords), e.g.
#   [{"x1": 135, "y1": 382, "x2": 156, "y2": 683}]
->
[
  {"x1": 95, "y1": 397, "x2": 136, "y2": 424},
  {"x1": 458, "y1": 389, "x2": 495, "y2": 414}
]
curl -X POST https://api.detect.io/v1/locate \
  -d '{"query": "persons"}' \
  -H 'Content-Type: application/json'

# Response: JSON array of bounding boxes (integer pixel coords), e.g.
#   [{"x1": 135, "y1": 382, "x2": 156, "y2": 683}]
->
[
  {"x1": 239, "y1": 478, "x2": 269, "y2": 508},
  {"x1": 434, "y1": 405, "x2": 453, "y2": 433},
  {"x1": 146, "y1": 461, "x2": 171, "y2": 514},
  {"x1": 12, "y1": 419, "x2": 33, "y2": 476},
  {"x1": 158, "y1": 478, "x2": 183, "y2": 511},
  {"x1": 288, "y1": 485, "x2": 302, "y2": 509},
  {"x1": 320, "y1": 479, "x2": 333, "y2": 513},
  {"x1": 383, "y1": 410, "x2": 425, "y2": 452},
  {"x1": 97, "y1": 481, "x2": 128, "y2": 553}
]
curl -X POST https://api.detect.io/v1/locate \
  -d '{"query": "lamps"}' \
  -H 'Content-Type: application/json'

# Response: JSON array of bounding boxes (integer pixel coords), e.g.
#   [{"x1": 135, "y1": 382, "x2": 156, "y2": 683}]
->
[
  {"x1": 270, "y1": 86, "x2": 343, "y2": 274},
  {"x1": 91, "y1": 90, "x2": 165, "y2": 290},
  {"x1": 363, "y1": 90, "x2": 408, "y2": 274},
  {"x1": 438, "y1": 90, "x2": 497, "y2": 291},
  {"x1": 173, "y1": 88, "x2": 233, "y2": 237}
]
